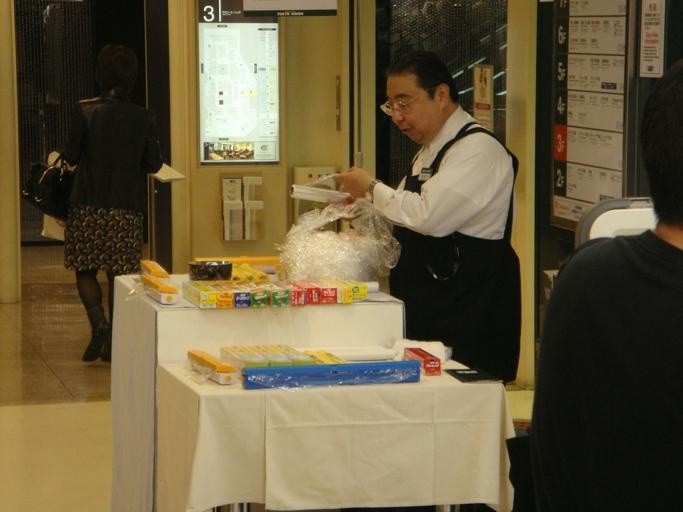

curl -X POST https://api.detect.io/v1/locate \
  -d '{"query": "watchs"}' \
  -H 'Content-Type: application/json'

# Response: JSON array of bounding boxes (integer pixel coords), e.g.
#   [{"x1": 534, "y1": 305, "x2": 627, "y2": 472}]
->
[{"x1": 365, "y1": 178, "x2": 381, "y2": 203}]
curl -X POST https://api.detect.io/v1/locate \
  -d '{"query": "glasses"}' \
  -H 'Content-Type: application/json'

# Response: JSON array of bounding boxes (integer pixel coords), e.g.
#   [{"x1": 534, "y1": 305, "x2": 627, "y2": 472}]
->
[{"x1": 380, "y1": 87, "x2": 429, "y2": 117}]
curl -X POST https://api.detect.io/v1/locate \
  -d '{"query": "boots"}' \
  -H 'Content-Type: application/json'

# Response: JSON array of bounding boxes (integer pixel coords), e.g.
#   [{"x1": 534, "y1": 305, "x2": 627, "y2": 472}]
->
[{"x1": 82, "y1": 305, "x2": 112, "y2": 362}]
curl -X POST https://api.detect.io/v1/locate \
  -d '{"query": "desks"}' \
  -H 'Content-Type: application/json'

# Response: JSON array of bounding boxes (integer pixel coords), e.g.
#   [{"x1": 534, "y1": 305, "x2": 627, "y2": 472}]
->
[
  {"x1": 110, "y1": 274, "x2": 404, "y2": 512},
  {"x1": 158, "y1": 348, "x2": 505, "y2": 512}
]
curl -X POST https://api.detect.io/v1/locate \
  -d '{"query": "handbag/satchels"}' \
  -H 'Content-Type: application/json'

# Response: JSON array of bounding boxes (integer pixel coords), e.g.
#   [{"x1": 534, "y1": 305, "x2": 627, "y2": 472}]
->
[{"x1": 22, "y1": 162, "x2": 75, "y2": 220}]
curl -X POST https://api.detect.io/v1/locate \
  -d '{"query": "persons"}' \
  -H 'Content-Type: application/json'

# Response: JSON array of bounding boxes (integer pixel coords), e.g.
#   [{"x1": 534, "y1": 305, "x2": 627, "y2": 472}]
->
[
  {"x1": 530, "y1": 57, "x2": 683, "y2": 512},
  {"x1": 333, "y1": 49, "x2": 520, "y2": 382},
  {"x1": 60, "y1": 43, "x2": 163, "y2": 363}
]
